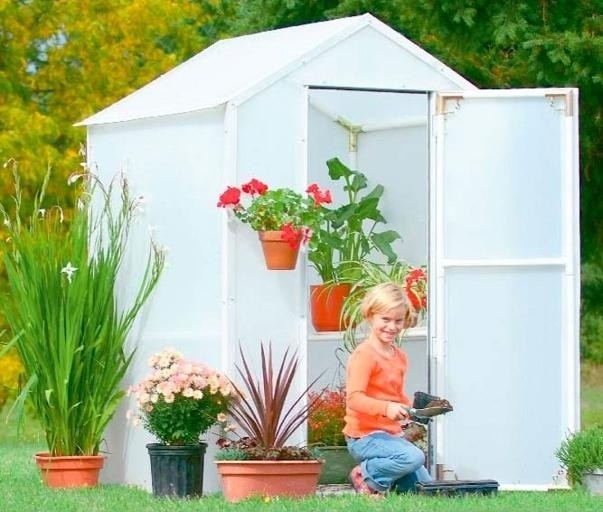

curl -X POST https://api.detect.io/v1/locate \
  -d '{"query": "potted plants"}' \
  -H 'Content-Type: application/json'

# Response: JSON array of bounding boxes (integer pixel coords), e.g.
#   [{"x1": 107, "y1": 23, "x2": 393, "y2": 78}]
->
[
  {"x1": 310, "y1": 157, "x2": 427, "y2": 331},
  {"x1": 307, "y1": 389, "x2": 363, "y2": 487},
  {"x1": 557, "y1": 421, "x2": 603, "y2": 495}
]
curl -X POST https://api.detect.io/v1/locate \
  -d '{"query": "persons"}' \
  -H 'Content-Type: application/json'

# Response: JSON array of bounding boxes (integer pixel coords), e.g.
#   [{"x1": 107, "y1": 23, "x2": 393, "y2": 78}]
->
[{"x1": 339, "y1": 278, "x2": 434, "y2": 501}]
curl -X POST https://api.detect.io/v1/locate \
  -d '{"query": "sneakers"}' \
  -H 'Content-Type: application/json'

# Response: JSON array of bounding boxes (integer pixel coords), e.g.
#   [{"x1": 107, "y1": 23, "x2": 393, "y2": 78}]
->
[{"x1": 348, "y1": 466, "x2": 381, "y2": 499}]
